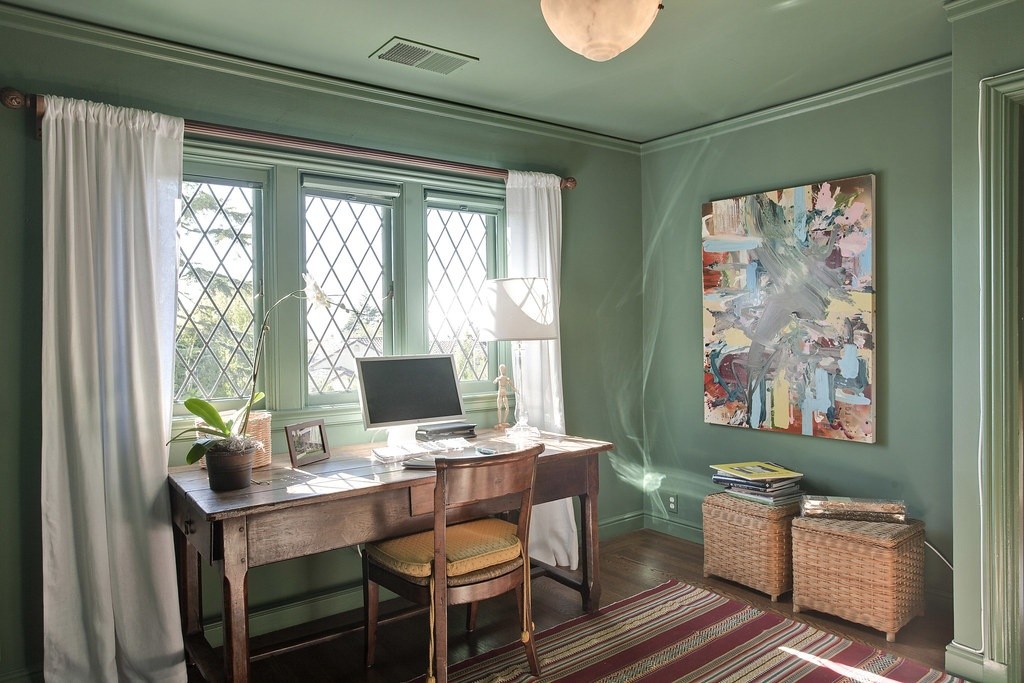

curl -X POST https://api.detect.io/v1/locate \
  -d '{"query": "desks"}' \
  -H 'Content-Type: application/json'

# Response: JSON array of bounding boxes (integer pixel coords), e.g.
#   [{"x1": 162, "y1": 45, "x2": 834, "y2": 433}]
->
[{"x1": 166, "y1": 423, "x2": 614, "y2": 683}]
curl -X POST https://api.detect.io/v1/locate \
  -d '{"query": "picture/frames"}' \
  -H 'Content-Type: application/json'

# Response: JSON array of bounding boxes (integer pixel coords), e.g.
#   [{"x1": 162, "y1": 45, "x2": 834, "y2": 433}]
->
[
  {"x1": 703, "y1": 173, "x2": 878, "y2": 444},
  {"x1": 284, "y1": 419, "x2": 330, "y2": 468}
]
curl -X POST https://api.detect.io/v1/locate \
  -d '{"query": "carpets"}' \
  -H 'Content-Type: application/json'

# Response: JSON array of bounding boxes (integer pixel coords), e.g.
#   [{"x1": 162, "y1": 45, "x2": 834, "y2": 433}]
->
[{"x1": 399, "y1": 578, "x2": 974, "y2": 683}]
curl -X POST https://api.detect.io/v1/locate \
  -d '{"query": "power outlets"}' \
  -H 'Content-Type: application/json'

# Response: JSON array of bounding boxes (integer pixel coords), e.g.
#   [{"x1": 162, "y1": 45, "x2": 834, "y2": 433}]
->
[{"x1": 667, "y1": 494, "x2": 679, "y2": 514}]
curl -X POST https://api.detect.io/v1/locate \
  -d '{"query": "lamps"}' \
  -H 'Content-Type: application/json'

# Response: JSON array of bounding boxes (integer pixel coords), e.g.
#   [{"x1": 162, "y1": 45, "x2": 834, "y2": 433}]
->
[
  {"x1": 540, "y1": 0, "x2": 664, "y2": 64},
  {"x1": 479, "y1": 278, "x2": 558, "y2": 439}
]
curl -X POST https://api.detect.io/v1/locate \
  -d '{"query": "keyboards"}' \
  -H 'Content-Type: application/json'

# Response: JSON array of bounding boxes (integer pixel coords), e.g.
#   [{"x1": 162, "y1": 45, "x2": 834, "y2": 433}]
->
[{"x1": 373, "y1": 437, "x2": 474, "y2": 464}]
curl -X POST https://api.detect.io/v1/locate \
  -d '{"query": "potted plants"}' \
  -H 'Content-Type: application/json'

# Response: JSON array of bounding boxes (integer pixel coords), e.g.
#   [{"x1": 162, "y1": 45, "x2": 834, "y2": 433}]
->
[{"x1": 166, "y1": 390, "x2": 266, "y2": 492}]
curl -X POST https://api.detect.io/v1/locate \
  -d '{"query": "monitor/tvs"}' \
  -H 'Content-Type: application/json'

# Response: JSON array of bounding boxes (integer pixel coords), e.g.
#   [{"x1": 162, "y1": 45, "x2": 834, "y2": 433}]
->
[{"x1": 354, "y1": 354, "x2": 465, "y2": 447}]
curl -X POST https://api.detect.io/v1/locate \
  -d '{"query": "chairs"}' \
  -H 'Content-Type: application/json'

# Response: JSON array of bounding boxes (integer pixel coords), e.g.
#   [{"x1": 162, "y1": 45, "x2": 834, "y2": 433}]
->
[{"x1": 361, "y1": 443, "x2": 546, "y2": 683}]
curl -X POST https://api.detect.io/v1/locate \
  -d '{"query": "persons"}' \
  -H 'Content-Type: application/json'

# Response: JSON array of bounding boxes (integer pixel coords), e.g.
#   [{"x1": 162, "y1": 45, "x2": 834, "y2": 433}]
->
[{"x1": 494, "y1": 365, "x2": 519, "y2": 425}]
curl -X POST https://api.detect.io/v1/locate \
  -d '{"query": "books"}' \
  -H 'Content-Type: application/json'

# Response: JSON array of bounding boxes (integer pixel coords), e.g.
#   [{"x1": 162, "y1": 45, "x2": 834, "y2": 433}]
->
[{"x1": 708, "y1": 461, "x2": 804, "y2": 506}]
